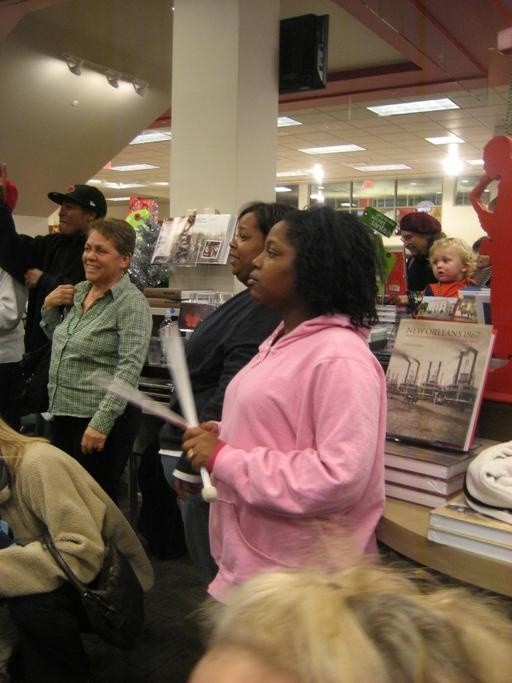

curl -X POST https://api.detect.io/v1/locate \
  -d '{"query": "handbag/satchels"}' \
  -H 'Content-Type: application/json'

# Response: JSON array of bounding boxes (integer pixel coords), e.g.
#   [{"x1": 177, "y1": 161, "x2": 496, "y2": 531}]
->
[
  {"x1": 22, "y1": 343, "x2": 49, "y2": 402},
  {"x1": 82, "y1": 544, "x2": 146, "y2": 651}
]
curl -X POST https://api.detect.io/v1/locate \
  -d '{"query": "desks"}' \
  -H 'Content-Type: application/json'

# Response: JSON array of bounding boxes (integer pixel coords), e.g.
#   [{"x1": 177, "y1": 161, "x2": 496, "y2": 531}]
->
[{"x1": 375, "y1": 436, "x2": 512, "y2": 600}]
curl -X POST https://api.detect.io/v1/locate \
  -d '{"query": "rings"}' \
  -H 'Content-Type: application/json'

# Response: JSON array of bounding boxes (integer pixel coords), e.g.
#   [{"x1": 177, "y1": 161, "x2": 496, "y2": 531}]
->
[{"x1": 187, "y1": 448, "x2": 195, "y2": 459}]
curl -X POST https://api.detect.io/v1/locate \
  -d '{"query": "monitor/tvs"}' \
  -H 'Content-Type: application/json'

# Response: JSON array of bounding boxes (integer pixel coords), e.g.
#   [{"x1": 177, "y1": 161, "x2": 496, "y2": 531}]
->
[{"x1": 279, "y1": 13, "x2": 329, "y2": 94}]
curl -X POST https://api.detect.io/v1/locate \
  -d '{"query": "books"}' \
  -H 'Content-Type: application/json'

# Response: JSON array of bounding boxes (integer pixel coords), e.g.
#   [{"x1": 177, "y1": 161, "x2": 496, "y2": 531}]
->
[{"x1": 365, "y1": 297, "x2": 512, "y2": 564}]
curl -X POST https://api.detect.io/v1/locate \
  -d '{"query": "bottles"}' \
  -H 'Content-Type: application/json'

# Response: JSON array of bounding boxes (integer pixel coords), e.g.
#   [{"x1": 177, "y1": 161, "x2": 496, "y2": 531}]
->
[{"x1": 157, "y1": 310, "x2": 176, "y2": 368}]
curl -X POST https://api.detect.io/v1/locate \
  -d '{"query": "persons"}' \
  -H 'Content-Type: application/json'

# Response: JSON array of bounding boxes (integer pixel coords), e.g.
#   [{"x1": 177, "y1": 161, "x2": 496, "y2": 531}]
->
[
  {"x1": 398, "y1": 210, "x2": 491, "y2": 298},
  {"x1": 0, "y1": 266, "x2": 26, "y2": 432},
  {"x1": 0, "y1": 419, "x2": 156, "y2": 683},
  {"x1": 38, "y1": 218, "x2": 154, "y2": 503},
  {"x1": 0, "y1": 161, "x2": 108, "y2": 409},
  {"x1": 183, "y1": 551, "x2": 512, "y2": 683},
  {"x1": 156, "y1": 202, "x2": 297, "y2": 580},
  {"x1": 183, "y1": 204, "x2": 388, "y2": 614}
]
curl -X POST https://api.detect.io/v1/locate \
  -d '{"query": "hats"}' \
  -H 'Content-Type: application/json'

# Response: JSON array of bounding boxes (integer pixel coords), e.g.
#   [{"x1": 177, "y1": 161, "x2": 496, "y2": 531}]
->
[
  {"x1": 48, "y1": 184, "x2": 107, "y2": 217},
  {"x1": 401, "y1": 212, "x2": 441, "y2": 234}
]
